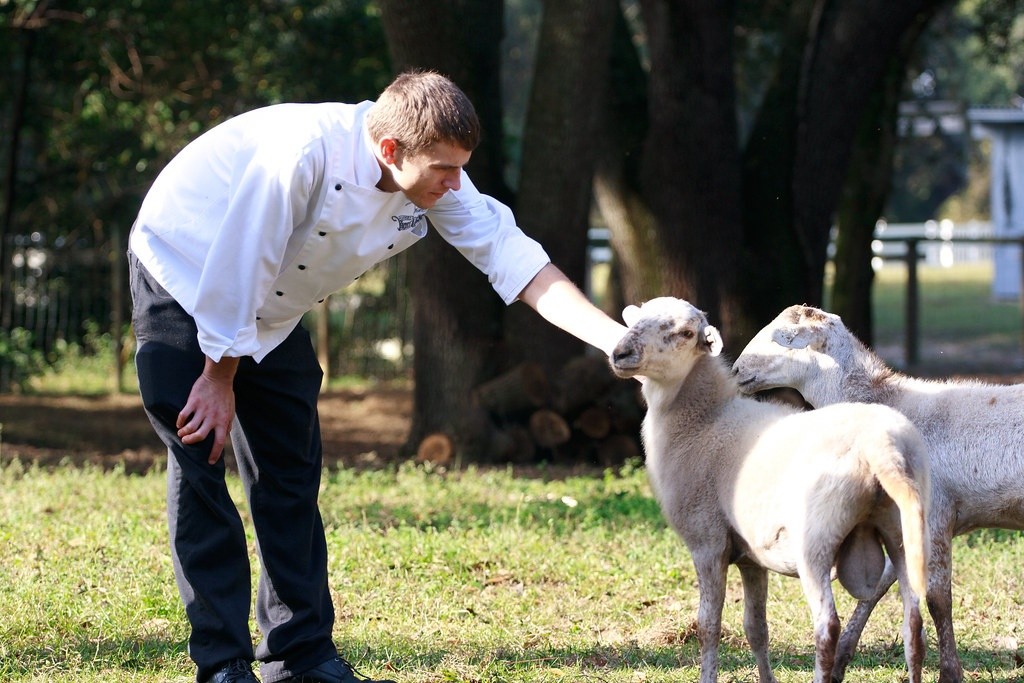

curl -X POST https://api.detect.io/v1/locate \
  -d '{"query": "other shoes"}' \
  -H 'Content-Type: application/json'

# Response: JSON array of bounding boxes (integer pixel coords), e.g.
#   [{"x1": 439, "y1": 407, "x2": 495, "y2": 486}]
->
[
  {"x1": 274, "y1": 653, "x2": 395, "y2": 683},
  {"x1": 201, "y1": 655, "x2": 262, "y2": 683}
]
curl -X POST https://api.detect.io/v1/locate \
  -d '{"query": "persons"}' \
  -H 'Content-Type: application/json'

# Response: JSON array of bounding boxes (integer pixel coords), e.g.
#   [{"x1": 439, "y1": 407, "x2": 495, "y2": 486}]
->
[{"x1": 125, "y1": 70, "x2": 634, "y2": 683}]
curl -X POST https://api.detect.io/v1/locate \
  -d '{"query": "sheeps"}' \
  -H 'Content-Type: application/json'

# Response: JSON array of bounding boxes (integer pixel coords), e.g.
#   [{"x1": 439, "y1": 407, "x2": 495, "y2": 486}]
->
[
  {"x1": 731, "y1": 302, "x2": 1024, "y2": 683},
  {"x1": 608, "y1": 297, "x2": 932, "y2": 683}
]
